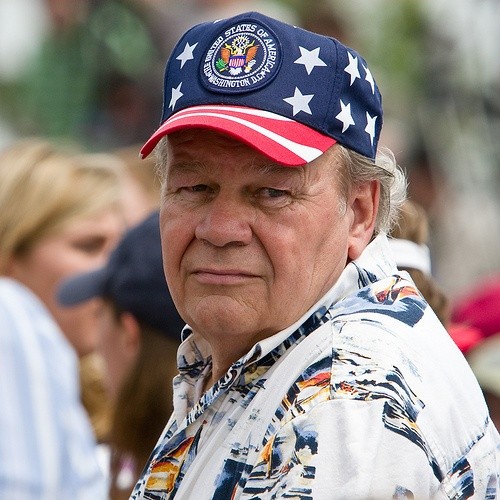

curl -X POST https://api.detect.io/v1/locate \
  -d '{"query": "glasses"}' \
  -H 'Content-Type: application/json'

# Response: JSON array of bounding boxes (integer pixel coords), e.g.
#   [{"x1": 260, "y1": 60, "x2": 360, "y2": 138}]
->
[{"x1": 70, "y1": 228, "x2": 106, "y2": 256}]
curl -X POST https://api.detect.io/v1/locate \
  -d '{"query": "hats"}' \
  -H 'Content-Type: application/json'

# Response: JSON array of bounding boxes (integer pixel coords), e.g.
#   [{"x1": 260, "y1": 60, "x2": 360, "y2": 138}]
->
[
  {"x1": 137, "y1": 12, "x2": 381, "y2": 166},
  {"x1": 57, "y1": 207, "x2": 190, "y2": 340}
]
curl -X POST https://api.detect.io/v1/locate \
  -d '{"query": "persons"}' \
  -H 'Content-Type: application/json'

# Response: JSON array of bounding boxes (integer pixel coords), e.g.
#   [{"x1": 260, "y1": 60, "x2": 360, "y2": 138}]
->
[
  {"x1": 137, "y1": 0, "x2": 499, "y2": 500},
  {"x1": 0, "y1": 105, "x2": 200, "y2": 499}
]
